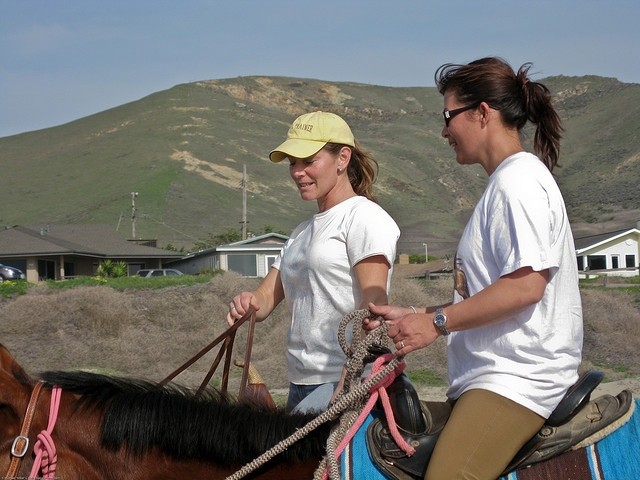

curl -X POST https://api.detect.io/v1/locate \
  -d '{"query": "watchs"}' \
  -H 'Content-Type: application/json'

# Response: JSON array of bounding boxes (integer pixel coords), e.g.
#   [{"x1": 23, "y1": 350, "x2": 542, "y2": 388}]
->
[{"x1": 433, "y1": 307, "x2": 451, "y2": 337}]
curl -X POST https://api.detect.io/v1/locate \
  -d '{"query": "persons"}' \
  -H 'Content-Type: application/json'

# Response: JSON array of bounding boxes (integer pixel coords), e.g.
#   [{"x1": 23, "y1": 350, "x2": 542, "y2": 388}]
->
[
  {"x1": 224, "y1": 109, "x2": 402, "y2": 415},
  {"x1": 360, "y1": 54, "x2": 586, "y2": 479}
]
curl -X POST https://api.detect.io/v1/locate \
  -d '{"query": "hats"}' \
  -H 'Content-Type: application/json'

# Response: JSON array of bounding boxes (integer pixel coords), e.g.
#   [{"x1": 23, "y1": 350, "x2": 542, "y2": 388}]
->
[{"x1": 268, "y1": 110, "x2": 355, "y2": 163}]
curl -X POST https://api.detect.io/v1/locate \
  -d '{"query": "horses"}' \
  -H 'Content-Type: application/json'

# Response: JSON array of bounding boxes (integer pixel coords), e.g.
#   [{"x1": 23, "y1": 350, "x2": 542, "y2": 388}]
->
[{"x1": 0, "y1": 344, "x2": 640, "y2": 480}]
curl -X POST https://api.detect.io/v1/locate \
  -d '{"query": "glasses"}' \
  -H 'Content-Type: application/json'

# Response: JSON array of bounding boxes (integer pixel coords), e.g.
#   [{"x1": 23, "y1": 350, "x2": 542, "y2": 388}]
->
[{"x1": 442, "y1": 96, "x2": 497, "y2": 126}]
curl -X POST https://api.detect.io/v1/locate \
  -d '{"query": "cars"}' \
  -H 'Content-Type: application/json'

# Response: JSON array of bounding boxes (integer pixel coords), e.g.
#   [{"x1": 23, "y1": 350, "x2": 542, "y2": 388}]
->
[
  {"x1": 135, "y1": 269, "x2": 183, "y2": 277},
  {"x1": 0, "y1": 263, "x2": 25, "y2": 283}
]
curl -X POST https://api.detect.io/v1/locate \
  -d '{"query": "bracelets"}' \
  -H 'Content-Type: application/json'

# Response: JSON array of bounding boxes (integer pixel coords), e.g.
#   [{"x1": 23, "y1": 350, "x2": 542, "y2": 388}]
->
[{"x1": 410, "y1": 304, "x2": 418, "y2": 314}]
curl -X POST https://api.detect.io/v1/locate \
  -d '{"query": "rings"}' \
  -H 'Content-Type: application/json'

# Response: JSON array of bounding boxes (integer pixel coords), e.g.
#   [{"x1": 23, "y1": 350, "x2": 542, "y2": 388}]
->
[
  {"x1": 399, "y1": 340, "x2": 406, "y2": 347},
  {"x1": 229, "y1": 306, "x2": 236, "y2": 311}
]
